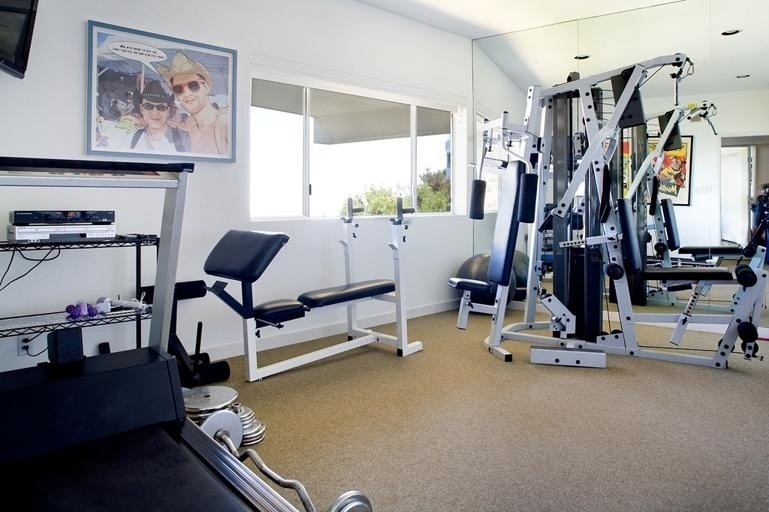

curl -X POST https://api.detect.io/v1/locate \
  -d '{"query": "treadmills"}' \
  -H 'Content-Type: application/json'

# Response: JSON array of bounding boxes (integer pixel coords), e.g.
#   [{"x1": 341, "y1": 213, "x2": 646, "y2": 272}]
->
[{"x1": 0, "y1": 156, "x2": 298, "y2": 512}]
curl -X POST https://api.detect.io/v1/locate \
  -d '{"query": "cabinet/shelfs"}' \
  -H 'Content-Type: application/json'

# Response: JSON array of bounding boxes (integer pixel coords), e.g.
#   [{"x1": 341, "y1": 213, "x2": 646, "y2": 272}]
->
[{"x1": 0, "y1": 155, "x2": 195, "y2": 372}]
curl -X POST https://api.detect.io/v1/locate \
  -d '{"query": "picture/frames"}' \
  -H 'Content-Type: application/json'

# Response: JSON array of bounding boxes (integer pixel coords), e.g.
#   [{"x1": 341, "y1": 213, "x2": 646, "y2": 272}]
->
[
  {"x1": 622, "y1": 135, "x2": 694, "y2": 206},
  {"x1": 87, "y1": 20, "x2": 237, "y2": 161}
]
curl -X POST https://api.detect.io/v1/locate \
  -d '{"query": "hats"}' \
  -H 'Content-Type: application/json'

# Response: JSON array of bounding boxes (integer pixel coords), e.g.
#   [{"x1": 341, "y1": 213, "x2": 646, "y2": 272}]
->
[
  {"x1": 169, "y1": 52, "x2": 213, "y2": 87},
  {"x1": 141, "y1": 81, "x2": 169, "y2": 103}
]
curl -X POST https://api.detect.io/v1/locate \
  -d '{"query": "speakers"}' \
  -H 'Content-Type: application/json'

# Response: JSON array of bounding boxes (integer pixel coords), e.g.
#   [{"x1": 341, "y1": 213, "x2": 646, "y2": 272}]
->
[{"x1": 47, "y1": 327, "x2": 84, "y2": 362}]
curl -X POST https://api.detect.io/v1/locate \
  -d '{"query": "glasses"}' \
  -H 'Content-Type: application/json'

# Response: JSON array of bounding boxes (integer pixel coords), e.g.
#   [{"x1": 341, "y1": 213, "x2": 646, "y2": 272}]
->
[
  {"x1": 140, "y1": 102, "x2": 168, "y2": 112},
  {"x1": 169, "y1": 80, "x2": 210, "y2": 95}
]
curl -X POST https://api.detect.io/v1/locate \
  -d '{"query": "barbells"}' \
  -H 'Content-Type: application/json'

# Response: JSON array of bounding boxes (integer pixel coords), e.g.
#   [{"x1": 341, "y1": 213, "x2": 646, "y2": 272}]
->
[{"x1": 200, "y1": 410, "x2": 372, "y2": 512}]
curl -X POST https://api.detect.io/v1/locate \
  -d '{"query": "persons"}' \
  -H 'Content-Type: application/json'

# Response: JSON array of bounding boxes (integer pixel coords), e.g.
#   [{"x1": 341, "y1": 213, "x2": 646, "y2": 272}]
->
[
  {"x1": 100, "y1": 86, "x2": 139, "y2": 116},
  {"x1": 747, "y1": 183, "x2": 769, "y2": 246},
  {"x1": 130, "y1": 78, "x2": 192, "y2": 153},
  {"x1": 158, "y1": 52, "x2": 228, "y2": 155}
]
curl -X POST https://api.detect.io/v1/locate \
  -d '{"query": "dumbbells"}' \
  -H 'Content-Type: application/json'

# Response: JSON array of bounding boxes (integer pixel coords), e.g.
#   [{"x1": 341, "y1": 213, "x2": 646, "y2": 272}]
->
[
  {"x1": 65, "y1": 305, "x2": 80, "y2": 319},
  {"x1": 86, "y1": 303, "x2": 97, "y2": 317}
]
